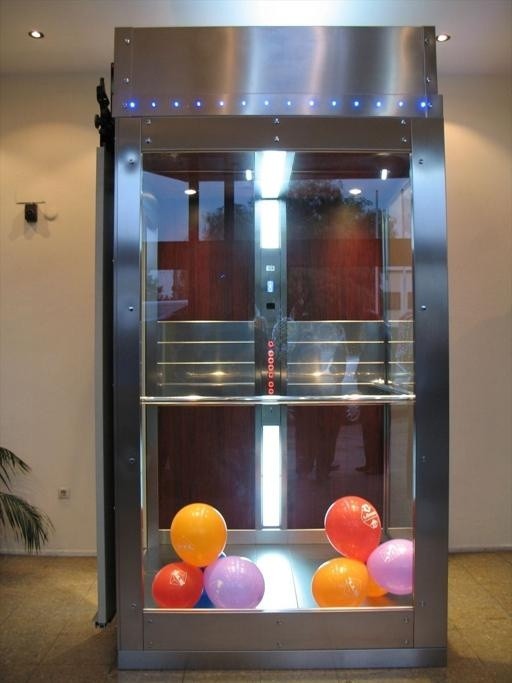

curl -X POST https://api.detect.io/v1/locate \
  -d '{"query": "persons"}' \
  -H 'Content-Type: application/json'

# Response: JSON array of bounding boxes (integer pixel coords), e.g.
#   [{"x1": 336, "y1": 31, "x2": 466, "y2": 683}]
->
[{"x1": 284, "y1": 265, "x2": 393, "y2": 498}]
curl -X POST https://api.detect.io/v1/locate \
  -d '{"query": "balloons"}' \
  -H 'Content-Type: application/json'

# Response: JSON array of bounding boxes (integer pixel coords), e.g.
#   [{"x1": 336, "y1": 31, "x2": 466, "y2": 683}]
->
[
  {"x1": 194, "y1": 551, "x2": 226, "y2": 607},
  {"x1": 152, "y1": 562, "x2": 204, "y2": 608},
  {"x1": 203, "y1": 555, "x2": 265, "y2": 609},
  {"x1": 324, "y1": 495, "x2": 382, "y2": 562},
  {"x1": 170, "y1": 502, "x2": 227, "y2": 567},
  {"x1": 312, "y1": 556, "x2": 368, "y2": 607},
  {"x1": 364, "y1": 561, "x2": 389, "y2": 595},
  {"x1": 367, "y1": 538, "x2": 413, "y2": 594}
]
